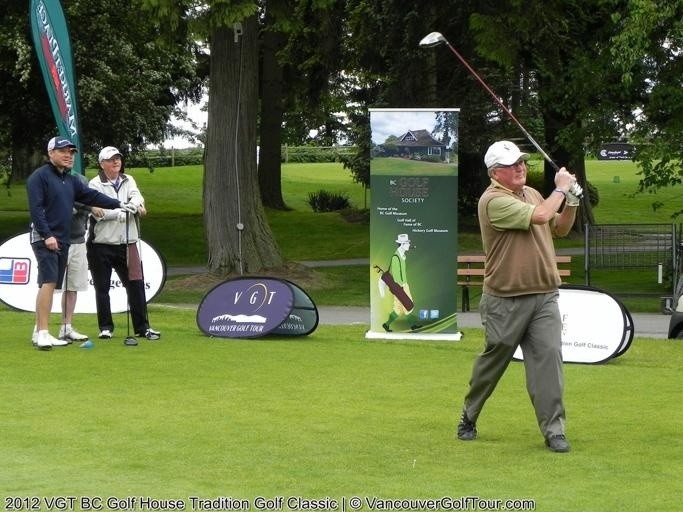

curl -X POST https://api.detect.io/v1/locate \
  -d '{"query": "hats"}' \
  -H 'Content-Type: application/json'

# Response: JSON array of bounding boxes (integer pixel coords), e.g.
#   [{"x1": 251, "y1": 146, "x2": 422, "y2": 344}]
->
[
  {"x1": 99, "y1": 146, "x2": 123, "y2": 162},
  {"x1": 484, "y1": 140, "x2": 529, "y2": 169},
  {"x1": 395, "y1": 234, "x2": 410, "y2": 243},
  {"x1": 48, "y1": 136, "x2": 76, "y2": 150}
]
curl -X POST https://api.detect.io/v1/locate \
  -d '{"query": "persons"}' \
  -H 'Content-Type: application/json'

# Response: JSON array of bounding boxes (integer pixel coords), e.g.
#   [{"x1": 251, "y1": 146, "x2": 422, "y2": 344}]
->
[
  {"x1": 381, "y1": 233, "x2": 423, "y2": 333},
  {"x1": 86, "y1": 144, "x2": 161, "y2": 341},
  {"x1": 23, "y1": 135, "x2": 136, "y2": 349},
  {"x1": 455, "y1": 140, "x2": 585, "y2": 454},
  {"x1": 29, "y1": 147, "x2": 104, "y2": 346}
]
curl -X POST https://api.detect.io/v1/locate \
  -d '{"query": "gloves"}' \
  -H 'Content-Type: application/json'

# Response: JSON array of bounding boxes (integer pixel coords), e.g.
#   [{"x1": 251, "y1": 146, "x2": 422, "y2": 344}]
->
[
  {"x1": 118, "y1": 201, "x2": 138, "y2": 223},
  {"x1": 565, "y1": 182, "x2": 583, "y2": 207}
]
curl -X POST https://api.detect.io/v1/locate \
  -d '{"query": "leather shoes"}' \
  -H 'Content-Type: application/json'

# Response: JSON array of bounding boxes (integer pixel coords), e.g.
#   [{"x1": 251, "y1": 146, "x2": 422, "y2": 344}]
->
[
  {"x1": 411, "y1": 325, "x2": 422, "y2": 330},
  {"x1": 383, "y1": 323, "x2": 392, "y2": 332}
]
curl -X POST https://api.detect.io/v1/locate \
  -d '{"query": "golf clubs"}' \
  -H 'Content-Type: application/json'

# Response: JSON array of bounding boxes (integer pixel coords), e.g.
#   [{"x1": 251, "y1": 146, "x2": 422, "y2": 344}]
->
[
  {"x1": 419, "y1": 31, "x2": 585, "y2": 200},
  {"x1": 122, "y1": 202, "x2": 138, "y2": 345},
  {"x1": 64, "y1": 261, "x2": 72, "y2": 343}
]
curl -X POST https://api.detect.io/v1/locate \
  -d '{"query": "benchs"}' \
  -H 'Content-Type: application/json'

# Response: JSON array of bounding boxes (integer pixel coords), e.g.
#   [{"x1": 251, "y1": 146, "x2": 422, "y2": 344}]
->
[{"x1": 457, "y1": 254, "x2": 575, "y2": 316}]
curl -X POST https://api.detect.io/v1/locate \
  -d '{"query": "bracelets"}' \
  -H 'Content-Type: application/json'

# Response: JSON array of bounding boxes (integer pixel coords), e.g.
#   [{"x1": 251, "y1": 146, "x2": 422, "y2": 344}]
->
[{"x1": 553, "y1": 186, "x2": 565, "y2": 196}]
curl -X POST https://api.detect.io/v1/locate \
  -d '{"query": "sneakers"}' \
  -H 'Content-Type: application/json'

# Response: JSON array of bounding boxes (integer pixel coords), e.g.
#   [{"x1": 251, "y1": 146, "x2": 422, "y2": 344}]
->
[
  {"x1": 32, "y1": 329, "x2": 88, "y2": 347},
  {"x1": 135, "y1": 328, "x2": 160, "y2": 337},
  {"x1": 546, "y1": 435, "x2": 568, "y2": 452},
  {"x1": 458, "y1": 410, "x2": 477, "y2": 440},
  {"x1": 99, "y1": 330, "x2": 112, "y2": 338}
]
